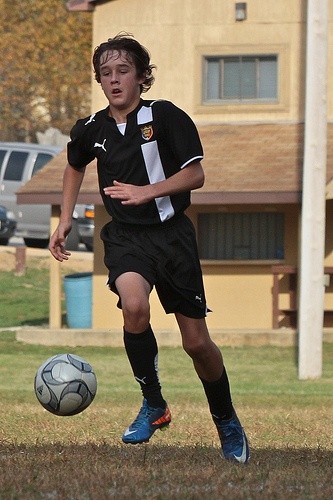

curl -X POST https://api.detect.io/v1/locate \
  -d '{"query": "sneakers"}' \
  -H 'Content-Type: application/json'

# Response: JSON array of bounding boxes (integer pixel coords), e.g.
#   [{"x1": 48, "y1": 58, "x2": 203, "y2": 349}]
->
[
  {"x1": 215, "y1": 409, "x2": 252, "y2": 466},
  {"x1": 120, "y1": 399, "x2": 173, "y2": 445}
]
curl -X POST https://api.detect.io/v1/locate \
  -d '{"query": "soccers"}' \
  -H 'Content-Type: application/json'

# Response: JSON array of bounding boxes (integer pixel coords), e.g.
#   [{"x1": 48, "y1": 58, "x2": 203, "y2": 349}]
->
[{"x1": 34, "y1": 353, "x2": 99, "y2": 417}]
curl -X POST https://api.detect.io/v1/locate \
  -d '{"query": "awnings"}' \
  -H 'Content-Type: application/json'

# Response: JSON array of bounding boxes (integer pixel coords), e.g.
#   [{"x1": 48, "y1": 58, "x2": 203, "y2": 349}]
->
[{"x1": 14, "y1": 118, "x2": 333, "y2": 329}]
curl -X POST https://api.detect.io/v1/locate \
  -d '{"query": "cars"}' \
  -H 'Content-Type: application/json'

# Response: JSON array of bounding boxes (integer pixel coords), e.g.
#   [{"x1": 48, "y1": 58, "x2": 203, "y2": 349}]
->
[{"x1": 0, "y1": 141, "x2": 94, "y2": 251}]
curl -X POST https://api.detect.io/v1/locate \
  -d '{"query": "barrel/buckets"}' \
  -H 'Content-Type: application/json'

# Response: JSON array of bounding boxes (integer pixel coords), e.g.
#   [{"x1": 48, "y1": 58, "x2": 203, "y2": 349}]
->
[{"x1": 65, "y1": 272, "x2": 93, "y2": 329}]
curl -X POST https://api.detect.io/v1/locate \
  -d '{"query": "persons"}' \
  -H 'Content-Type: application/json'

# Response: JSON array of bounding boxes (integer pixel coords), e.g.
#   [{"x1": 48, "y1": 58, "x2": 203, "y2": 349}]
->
[{"x1": 46, "y1": 30, "x2": 253, "y2": 468}]
